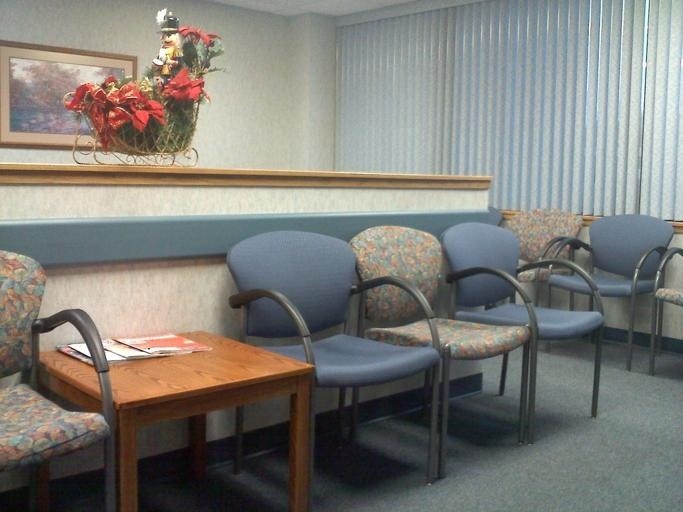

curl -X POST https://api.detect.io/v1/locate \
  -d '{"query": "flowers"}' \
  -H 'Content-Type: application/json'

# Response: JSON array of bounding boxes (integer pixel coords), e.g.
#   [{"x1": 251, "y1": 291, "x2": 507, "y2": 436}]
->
[{"x1": 66, "y1": 26, "x2": 228, "y2": 146}]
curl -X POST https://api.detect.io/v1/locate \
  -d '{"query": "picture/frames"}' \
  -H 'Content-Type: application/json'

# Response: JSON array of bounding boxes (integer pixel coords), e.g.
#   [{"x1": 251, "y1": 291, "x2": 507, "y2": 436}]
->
[{"x1": 0, "y1": 39, "x2": 138, "y2": 151}]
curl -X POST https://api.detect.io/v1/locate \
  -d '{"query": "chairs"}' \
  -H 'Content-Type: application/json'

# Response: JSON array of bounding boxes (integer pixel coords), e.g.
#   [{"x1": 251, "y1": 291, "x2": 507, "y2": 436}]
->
[
  {"x1": 482, "y1": 208, "x2": 584, "y2": 311},
  {"x1": 347, "y1": 224, "x2": 532, "y2": 480},
  {"x1": 224, "y1": 230, "x2": 441, "y2": 512},
  {"x1": 0, "y1": 248, "x2": 117, "y2": 512},
  {"x1": 647, "y1": 247, "x2": 683, "y2": 377},
  {"x1": 545, "y1": 214, "x2": 674, "y2": 371},
  {"x1": 440, "y1": 221, "x2": 605, "y2": 446}
]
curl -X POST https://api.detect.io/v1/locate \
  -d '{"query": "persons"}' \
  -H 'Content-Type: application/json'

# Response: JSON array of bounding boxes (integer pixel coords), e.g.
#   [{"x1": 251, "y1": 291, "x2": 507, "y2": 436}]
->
[{"x1": 142, "y1": 6, "x2": 184, "y2": 85}]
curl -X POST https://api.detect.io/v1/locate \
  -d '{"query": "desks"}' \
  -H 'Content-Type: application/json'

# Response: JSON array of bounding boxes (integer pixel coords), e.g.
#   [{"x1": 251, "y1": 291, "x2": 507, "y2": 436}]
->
[{"x1": 38, "y1": 329, "x2": 317, "y2": 512}]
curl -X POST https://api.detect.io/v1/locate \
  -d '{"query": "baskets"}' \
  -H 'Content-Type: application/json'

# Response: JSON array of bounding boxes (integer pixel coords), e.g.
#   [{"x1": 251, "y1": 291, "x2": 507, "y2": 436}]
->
[{"x1": 61, "y1": 80, "x2": 206, "y2": 167}]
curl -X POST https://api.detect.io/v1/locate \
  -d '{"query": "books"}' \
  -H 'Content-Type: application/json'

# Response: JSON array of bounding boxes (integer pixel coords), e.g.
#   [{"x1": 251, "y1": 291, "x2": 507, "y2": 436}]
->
[{"x1": 55, "y1": 331, "x2": 214, "y2": 368}]
